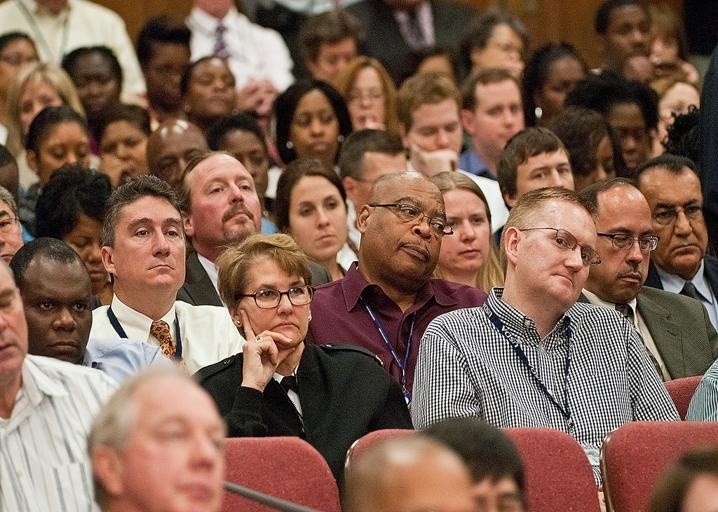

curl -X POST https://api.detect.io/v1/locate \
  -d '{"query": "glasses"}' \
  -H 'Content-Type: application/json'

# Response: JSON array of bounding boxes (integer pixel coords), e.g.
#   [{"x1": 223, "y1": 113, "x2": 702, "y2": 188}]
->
[
  {"x1": 597, "y1": 232, "x2": 660, "y2": 251},
  {"x1": 369, "y1": 202, "x2": 455, "y2": 237},
  {"x1": 518, "y1": 227, "x2": 603, "y2": 267},
  {"x1": 237, "y1": 284, "x2": 316, "y2": 310}
]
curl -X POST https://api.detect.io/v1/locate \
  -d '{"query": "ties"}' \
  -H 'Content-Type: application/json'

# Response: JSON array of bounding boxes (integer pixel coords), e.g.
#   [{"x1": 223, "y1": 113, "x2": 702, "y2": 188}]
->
[
  {"x1": 280, "y1": 375, "x2": 306, "y2": 431},
  {"x1": 406, "y1": 5, "x2": 427, "y2": 48},
  {"x1": 679, "y1": 283, "x2": 699, "y2": 299},
  {"x1": 150, "y1": 319, "x2": 178, "y2": 361},
  {"x1": 213, "y1": 24, "x2": 230, "y2": 64},
  {"x1": 615, "y1": 304, "x2": 667, "y2": 383}
]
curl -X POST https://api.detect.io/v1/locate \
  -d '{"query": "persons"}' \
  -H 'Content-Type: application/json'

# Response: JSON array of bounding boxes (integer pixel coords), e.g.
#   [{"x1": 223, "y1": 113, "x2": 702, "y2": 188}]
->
[
  {"x1": 300, "y1": 170, "x2": 505, "y2": 415},
  {"x1": 1, "y1": 1, "x2": 718, "y2": 182},
  {"x1": 28, "y1": 166, "x2": 113, "y2": 306},
  {"x1": 344, "y1": 418, "x2": 718, "y2": 512},
  {"x1": 1, "y1": 187, "x2": 24, "y2": 265},
  {"x1": 633, "y1": 153, "x2": 718, "y2": 330},
  {"x1": 396, "y1": 74, "x2": 467, "y2": 177},
  {"x1": 87, "y1": 370, "x2": 223, "y2": 512},
  {"x1": 144, "y1": 119, "x2": 211, "y2": 199},
  {"x1": 87, "y1": 176, "x2": 245, "y2": 373},
  {"x1": 0, "y1": 260, "x2": 118, "y2": 512},
  {"x1": 9, "y1": 238, "x2": 171, "y2": 380},
  {"x1": 563, "y1": 74, "x2": 661, "y2": 174},
  {"x1": 273, "y1": 159, "x2": 352, "y2": 282},
  {"x1": 574, "y1": 179, "x2": 718, "y2": 381},
  {"x1": 340, "y1": 56, "x2": 399, "y2": 133},
  {"x1": 406, "y1": 189, "x2": 682, "y2": 491},
  {"x1": 275, "y1": 81, "x2": 348, "y2": 162},
  {"x1": 93, "y1": 100, "x2": 152, "y2": 188},
  {"x1": 190, "y1": 236, "x2": 412, "y2": 432},
  {"x1": 458, "y1": 68, "x2": 532, "y2": 176},
  {"x1": 25, "y1": 106, "x2": 92, "y2": 215},
  {"x1": 498, "y1": 129, "x2": 575, "y2": 204},
  {"x1": 176, "y1": 149, "x2": 331, "y2": 307},
  {"x1": 429, "y1": 174, "x2": 503, "y2": 289}
]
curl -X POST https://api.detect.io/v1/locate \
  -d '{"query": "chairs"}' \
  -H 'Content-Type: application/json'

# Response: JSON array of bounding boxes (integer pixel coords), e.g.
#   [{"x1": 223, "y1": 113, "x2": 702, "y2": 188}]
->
[
  {"x1": 214, "y1": 435, "x2": 342, "y2": 512},
  {"x1": 660, "y1": 375, "x2": 704, "y2": 422},
  {"x1": 344, "y1": 426, "x2": 601, "y2": 512},
  {"x1": 599, "y1": 421, "x2": 718, "y2": 512}
]
